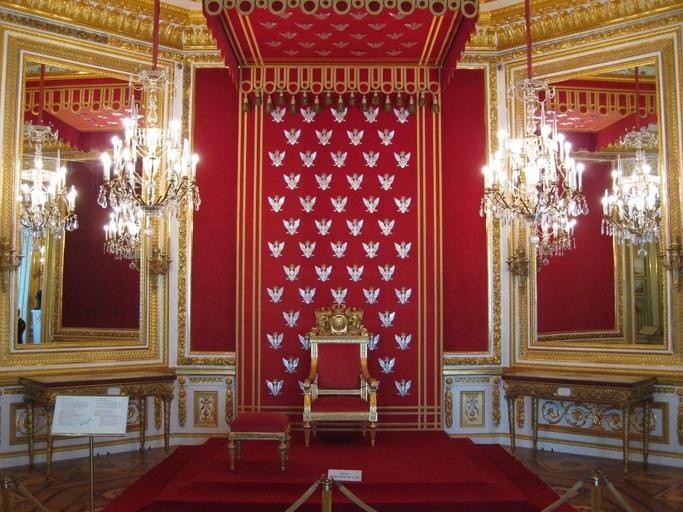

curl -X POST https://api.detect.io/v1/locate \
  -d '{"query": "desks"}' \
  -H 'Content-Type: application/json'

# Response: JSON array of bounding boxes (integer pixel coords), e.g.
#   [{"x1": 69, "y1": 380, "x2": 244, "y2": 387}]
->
[
  {"x1": 19, "y1": 370, "x2": 177, "y2": 477},
  {"x1": 500, "y1": 373, "x2": 657, "y2": 475}
]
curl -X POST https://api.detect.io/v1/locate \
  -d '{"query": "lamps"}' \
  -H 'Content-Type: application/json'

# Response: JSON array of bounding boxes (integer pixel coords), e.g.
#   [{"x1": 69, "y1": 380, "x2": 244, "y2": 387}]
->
[
  {"x1": 599, "y1": 66, "x2": 661, "y2": 258},
  {"x1": 102, "y1": 204, "x2": 144, "y2": 269},
  {"x1": 479, "y1": 1, "x2": 586, "y2": 265},
  {"x1": 96, "y1": 0, "x2": 204, "y2": 210},
  {"x1": 18, "y1": 62, "x2": 80, "y2": 252}
]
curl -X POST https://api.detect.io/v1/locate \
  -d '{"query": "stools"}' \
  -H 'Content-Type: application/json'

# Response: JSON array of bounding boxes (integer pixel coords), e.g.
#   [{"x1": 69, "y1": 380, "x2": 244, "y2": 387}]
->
[{"x1": 227, "y1": 411, "x2": 291, "y2": 472}]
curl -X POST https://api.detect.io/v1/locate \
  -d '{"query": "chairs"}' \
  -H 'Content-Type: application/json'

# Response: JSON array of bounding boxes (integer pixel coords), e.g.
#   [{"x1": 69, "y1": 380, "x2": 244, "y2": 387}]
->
[{"x1": 303, "y1": 303, "x2": 378, "y2": 448}]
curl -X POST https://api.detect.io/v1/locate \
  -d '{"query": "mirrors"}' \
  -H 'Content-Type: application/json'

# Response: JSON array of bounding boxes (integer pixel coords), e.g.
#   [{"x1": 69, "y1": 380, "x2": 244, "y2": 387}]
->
[
  {"x1": 505, "y1": 27, "x2": 683, "y2": 376},
  {"x1": 0, "y1": 34, "x2": 164, "y2": 367}
]
